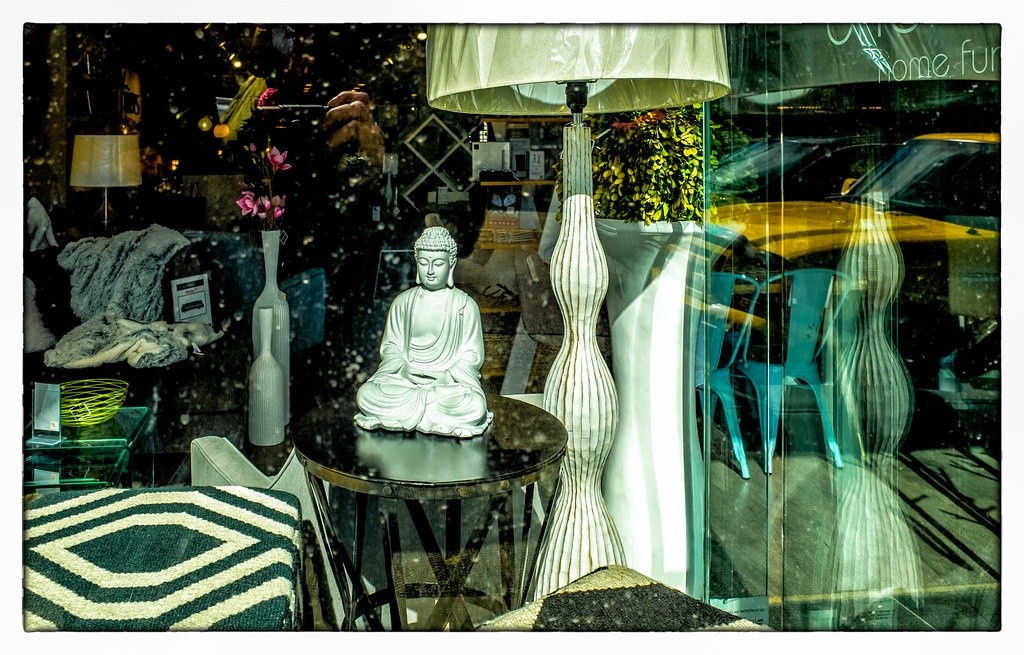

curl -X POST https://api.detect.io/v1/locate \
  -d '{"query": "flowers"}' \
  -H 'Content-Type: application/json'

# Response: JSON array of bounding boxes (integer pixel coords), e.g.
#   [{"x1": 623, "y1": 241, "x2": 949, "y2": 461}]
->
[{"x1": 236, "y1": 137, "x2": 292, "y2": 230}]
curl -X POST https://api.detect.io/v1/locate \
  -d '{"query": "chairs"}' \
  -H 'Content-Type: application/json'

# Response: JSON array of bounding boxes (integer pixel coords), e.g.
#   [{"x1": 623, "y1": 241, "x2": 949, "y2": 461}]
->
[
  {"x1": 737, "y1": 269, "x2": 850, "y2": 474},
  {"x1": 695, "y1": 273, "x2": 759, "y2": 480}
]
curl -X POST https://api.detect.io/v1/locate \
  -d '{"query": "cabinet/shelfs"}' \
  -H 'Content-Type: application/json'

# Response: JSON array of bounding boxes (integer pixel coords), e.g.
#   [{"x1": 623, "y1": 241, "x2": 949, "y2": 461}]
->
[{"x1": 457, "y1": 118, "x2": 575, "y2": 313}]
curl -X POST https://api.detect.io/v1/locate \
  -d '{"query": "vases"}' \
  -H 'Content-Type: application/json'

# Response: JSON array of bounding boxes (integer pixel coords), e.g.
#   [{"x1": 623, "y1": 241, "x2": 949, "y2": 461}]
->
[
  {"x1": 249, "y1": 307, "x2": 284, "y2": 447},
  {"x1": 252, "y1": 230, "x2": 290, "y2": 425}
]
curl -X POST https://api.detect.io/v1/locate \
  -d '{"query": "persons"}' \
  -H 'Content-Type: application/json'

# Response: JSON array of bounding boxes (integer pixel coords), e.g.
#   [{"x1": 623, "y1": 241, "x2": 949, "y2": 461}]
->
[
  {"x1": 23, "y1": 187, "x2": 60, "y2": 252},
  {"x1": 325, "y1": 90, "x2": 386, "y2": 187},
  {"x1": 356, "y1": 226, "x2": 494, "y2": 436}
]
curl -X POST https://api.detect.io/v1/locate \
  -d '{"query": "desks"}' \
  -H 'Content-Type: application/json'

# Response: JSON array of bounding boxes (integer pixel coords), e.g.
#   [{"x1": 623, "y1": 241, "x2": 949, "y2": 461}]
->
[{"x1": 291, "y1": 393, "x2": 568, "y2": 632}]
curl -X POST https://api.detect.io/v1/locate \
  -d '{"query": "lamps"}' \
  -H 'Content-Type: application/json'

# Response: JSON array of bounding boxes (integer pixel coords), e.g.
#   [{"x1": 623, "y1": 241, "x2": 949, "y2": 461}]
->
[
  {"x1": 426, "y1": 25, "x2": 730, "y2": 602},
  {"x1": 70, "y1": 136, "x2": 142, "y2": 229},
  {"x1": 725, "y1": 24, "x2": 1000, "y2": 617}
]
